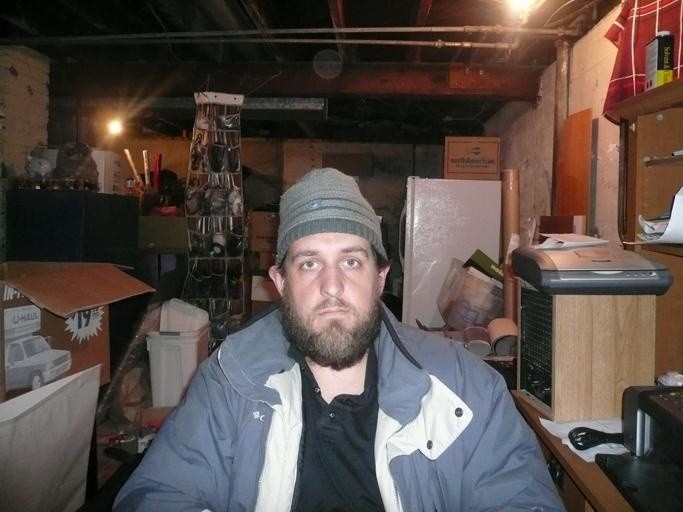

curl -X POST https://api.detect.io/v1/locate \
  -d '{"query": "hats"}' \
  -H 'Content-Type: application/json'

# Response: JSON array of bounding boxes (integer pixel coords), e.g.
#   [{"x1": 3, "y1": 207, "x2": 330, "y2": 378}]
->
[{"x1": 274, "y1": 167, "x2": 389, "y2": 267}]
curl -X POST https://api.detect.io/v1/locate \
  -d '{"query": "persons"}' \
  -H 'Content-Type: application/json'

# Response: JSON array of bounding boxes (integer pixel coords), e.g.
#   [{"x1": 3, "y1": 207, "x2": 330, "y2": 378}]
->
[{"x1": 110, "y1": 165, "x2": 566, "y2": 512}]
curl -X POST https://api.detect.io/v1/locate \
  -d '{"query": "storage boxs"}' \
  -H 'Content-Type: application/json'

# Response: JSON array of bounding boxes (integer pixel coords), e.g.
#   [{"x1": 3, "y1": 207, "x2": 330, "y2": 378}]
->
[
  {"x1": 436, "y1": 257, "x2": 504, "y2": 329},
  {"x1": 247, "y1": 210, "x2": 283, "y2": 318},
  {"x1": 1, "y1": 261, "x2": 157, "y2": 402},
  {"x1": 444, "y1": 137, "x2": 501, "y2": 179}
]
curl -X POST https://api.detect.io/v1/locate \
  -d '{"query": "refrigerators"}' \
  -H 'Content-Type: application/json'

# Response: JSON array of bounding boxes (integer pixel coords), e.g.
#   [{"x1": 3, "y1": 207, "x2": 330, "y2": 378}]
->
[{"x1": 397, "y1": 177, "x2": 501, "y2": 329}]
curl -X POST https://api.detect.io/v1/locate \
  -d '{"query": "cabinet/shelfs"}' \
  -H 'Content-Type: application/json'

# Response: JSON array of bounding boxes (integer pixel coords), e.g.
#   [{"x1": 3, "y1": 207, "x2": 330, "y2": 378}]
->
[{"x1": 511, "y1": 75, "x2": 683, "y2": 512}]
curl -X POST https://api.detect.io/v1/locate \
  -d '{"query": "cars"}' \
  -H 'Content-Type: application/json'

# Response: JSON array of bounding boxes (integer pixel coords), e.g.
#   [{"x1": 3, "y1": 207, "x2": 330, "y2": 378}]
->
[{"x1": 4, "y1": 334, "x2": 73, "y2": 393}]
